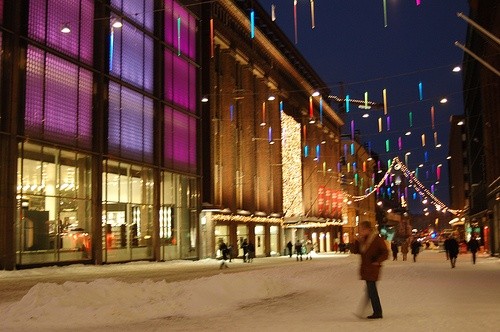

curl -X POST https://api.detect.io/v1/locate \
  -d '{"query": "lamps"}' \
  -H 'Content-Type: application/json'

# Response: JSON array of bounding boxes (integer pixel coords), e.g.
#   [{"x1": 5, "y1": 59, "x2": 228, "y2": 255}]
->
[
  {"x1": 201, "y1": 95, "x2": 208, "y2": 102},
  {"x1": 60, "y1": 22, "x2": 71, "y2": 33},
  {"x1": 110, "y1": 18, "x2": 122, "y2": 29},
  {"x1": 267, "y1": 94, "x2": 275, "y2": 101},
  {"x1": 311, "y1": 89, "x2": 320, "y2": 96}
]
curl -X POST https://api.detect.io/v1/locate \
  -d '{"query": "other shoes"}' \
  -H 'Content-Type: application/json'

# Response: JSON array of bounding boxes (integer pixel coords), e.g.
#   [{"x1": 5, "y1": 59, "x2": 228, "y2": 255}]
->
[
  {"x1": 297, "y1": 257, "x2": 299, "y2": 261},
  {"x1": 367, "y1": 315, "x2": 383, "y2": 319},
  {"x1": 300, "y1": 257, "x2": 302, "y2": 261}
]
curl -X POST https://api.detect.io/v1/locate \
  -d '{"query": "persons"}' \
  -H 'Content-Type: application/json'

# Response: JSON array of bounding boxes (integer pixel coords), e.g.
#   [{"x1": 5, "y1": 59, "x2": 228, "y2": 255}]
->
[
  {"x1": 343, "y1": 243, "x2": 346, "y2": 253},
  {"x1": 426, "y1": 241, "x2": 430, "y2": 250},
  {"x1": 469, "y1": 237, "x2": 480, "y2": 265},
  {"x1": 401, "y1": 240, "x2": 409, "y2": 262},
  {"x1": 219, "y1": 240, "x2": 229, "y2": 270},
  {"x1": 286, "y1": 241, "x2": 292, "y2": 258},
  {"x1": 246, "y1": 241, "x2": 255, "y2": 262},
  {"x1": 410, "y1": 239, "x2": 421, "y2": 262},
  {"x1": 334, "y1": 241, "x2": 337, "y2": 253},
  {"x1": 350, "y1": 221, "x2": 389, "y2": 319},
  {"x1": 338, "y1": 242, "x2": 342, "y2": 254},
  {"x1": 304, "y1": 242, "x2": 312, "y2": 260},
  {"x1": 228, "y1": 245, "x2": 233, "y2": 262},
  {"x1": 240, "y1": 238, "x2": 248, "y2": 262},
  {"x1": 447, "y1": 238, "x2": 459, "y2": 268},
  {"x1": 392, "y1": 241, "x2": 398, "y2": 261},
  {"x1": 444, "y1": 237, "x2": 450, "y2": 259},
  {"x1": 295, "y1": 240, "x2": 303, "y2": 261}
]
